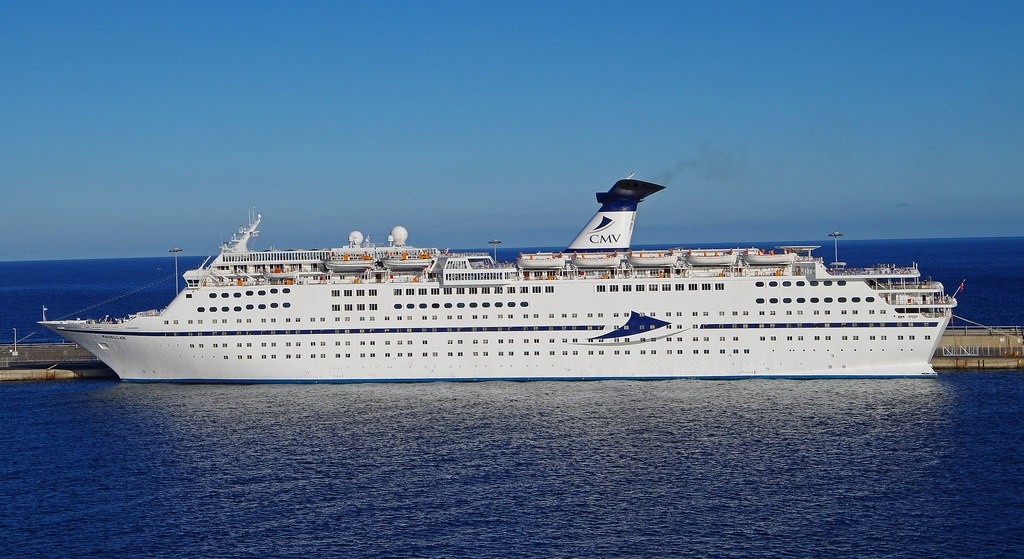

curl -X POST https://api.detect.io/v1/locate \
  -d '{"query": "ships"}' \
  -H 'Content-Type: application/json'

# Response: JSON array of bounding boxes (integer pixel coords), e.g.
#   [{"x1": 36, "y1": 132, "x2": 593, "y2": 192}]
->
[{"x1": 34, "y1": 171, "x2": 967, "y2": 382}]
